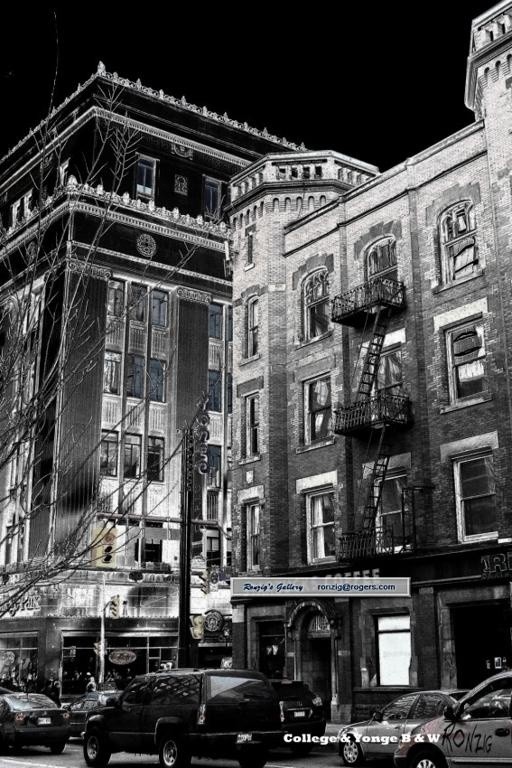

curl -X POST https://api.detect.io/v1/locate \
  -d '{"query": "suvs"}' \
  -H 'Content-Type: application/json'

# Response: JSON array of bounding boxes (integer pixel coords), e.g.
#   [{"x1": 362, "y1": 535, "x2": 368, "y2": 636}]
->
[{"x1": 84, "y1": 667, "x2": 285, "y2": 768}]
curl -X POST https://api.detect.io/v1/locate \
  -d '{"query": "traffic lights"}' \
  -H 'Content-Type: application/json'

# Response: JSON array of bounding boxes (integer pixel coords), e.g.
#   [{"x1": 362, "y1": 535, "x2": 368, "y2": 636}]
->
[{"x1": 101, "y1": 545, "x2": 112, "y2": 563}]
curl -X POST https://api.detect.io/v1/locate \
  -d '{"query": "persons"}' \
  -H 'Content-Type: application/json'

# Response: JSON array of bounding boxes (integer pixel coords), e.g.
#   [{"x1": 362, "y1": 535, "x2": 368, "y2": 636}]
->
[
  {"x1": 85, "y1": 681, "x2": 98, "y2": 696},
  {"x1": 40, "y1": 680, "x2": 54, "y2": 695},
  {"x1": 48, "y1": 680, "x2": 62, "y2": 706},
  {"x1": 86, "y1": 671, "x2": 96, "y2": 682}
]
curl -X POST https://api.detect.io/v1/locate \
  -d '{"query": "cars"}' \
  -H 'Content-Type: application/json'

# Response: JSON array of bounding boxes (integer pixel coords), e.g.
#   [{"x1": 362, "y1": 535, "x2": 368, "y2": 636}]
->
[
  {"x1": 63, "y1": 690, "x2": 124, "y2": 737},
  {"x1": 338, "y1": 688, "x2": 469, "y2": 766},
  {"x1": 393, "y1": 670, "x2": 512, "y2": 768},
  {"x1": 269, "y1": 679, "x2": 326, "y2": 758},
  {"x1": 0, "y1": 692, "x2": 72, "y2": 756}
]
